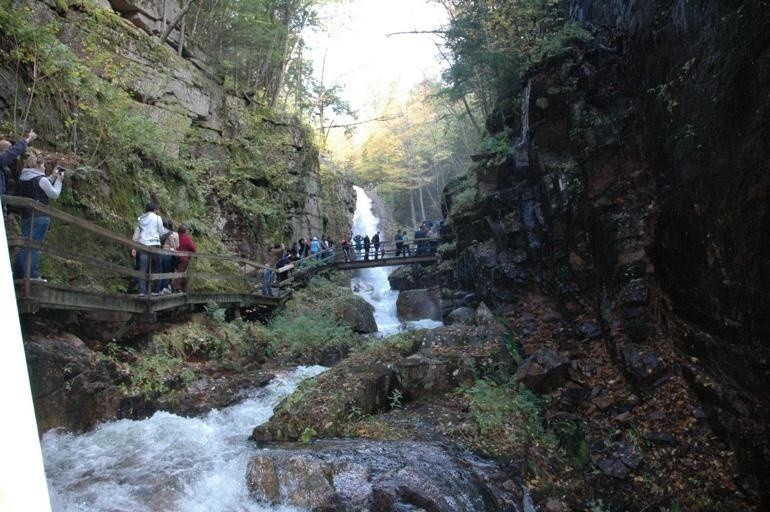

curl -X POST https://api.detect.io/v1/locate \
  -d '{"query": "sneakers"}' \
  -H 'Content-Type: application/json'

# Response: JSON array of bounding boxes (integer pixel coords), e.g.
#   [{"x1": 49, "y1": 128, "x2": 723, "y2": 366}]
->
[{"x1": 138, "y1": 284, "x2": 172, "y2": 297}]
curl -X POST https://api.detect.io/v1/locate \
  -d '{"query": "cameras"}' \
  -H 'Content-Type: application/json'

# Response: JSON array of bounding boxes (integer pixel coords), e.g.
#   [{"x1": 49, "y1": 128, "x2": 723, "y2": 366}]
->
[{"x1": 58, "y1": 167, "x2": 65, "y2": 176}]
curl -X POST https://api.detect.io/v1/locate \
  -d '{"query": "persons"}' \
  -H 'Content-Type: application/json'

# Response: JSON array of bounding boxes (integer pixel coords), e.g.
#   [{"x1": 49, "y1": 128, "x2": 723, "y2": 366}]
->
[
  {"x1": 127, "y1": 202, "x2": 199, "y2": 297},
  {"x1": 0, "y1": 128, "x2": 39, "y2": 259},
  {"x1": 261, "y1": 217, "x2": 445, "y2": 298},
  {"x1": 12, "y1": 152, "x2": 66, "y2": 284}
]
